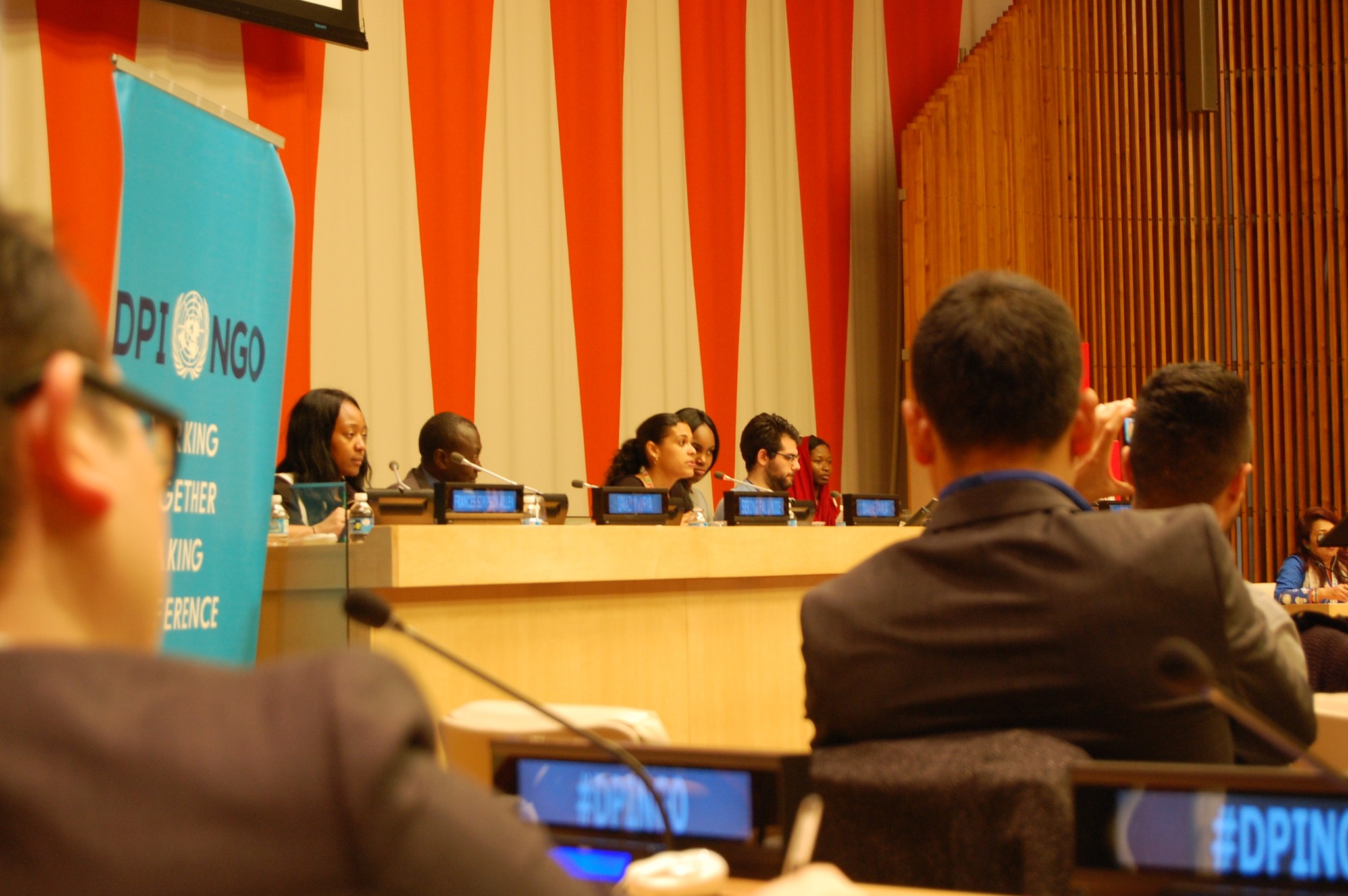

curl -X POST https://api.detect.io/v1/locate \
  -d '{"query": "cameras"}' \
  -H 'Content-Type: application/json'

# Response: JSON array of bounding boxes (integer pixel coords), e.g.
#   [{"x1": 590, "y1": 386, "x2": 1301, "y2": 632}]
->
[{"x1": 1122, "y1": 415, "x2": 1139, "y2": 444}]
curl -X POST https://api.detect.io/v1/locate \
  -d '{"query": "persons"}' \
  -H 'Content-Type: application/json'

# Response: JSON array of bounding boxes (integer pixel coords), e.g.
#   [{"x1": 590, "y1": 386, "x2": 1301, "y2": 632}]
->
[
  {"x1": 274, "y1": 389, "x2": 374, "y2": 541},
  {"x1": 785, "y1": 435, "x2": 840, "y2": 525},
  {"x1": 1072, "y1": 360, "x2": 1308, "y2": 686},
  {"x1": 384, "y1": 411, "x2": 482, "y2": 492},
  {"x1": 603, "y1": 407, "x2": 719, "y2": 527},
  {"x1": 800, "y1": 274, "x2": 1319, "y2": 767},
  {"x1": 1274, "y1": 506, "x2": 1348, "y2": 605},
  {"x1": 0, "y1": 210, "x2": 845, "y2": 896},
  {"x1": 713, "y1": 413, "x2": 802, "y2": 526}
]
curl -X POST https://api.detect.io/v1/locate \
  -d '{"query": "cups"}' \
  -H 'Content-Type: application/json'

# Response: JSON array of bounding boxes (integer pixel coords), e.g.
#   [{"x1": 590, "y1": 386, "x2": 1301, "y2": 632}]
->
[
  {"x1": 711, "y1": 521, "x2": 727, "y2": 526},
  {"x1": 811, "y1": 521, "x2": 825, "y2": 526},
  {"x1": 520, "y1": 494, "x2": 549, "y2": 524}
]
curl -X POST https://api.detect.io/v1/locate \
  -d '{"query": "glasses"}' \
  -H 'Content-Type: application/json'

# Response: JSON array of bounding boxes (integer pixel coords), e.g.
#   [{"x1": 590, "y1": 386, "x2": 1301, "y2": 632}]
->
[
  {"x1": 1309, "y1": 534, "x2": 1326, "y2": 542},
  {"x1": 4, "y1": 373, "x2": 184, "y2": 492},
  {"x1": 764, "y1": 448, "x2": 800, "y2": 464}
]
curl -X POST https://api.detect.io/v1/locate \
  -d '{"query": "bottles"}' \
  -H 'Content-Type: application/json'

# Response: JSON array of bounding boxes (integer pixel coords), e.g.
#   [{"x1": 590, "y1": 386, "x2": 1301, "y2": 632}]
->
[
  {"x1": 347, "y1": 493, "x2": 376, "y2": 542},
  {"x1": 835, "y1": 504, "x2": 846, "y2": 525},
  {"x1": 689, "y1": 508, "x2": 708, "y2": 526},
  {"x1": 786, "y1": 503, "x2": 798, "y2": 525},
  {"x1": 267, "y1": 495, "x2": 289, "y2": 546}
]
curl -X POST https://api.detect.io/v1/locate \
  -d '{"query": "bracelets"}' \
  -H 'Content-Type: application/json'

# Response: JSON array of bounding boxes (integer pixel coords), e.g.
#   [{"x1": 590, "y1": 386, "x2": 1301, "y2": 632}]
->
[{"x1": 312, "y1": 525, "x2": 315, "y2": 534}]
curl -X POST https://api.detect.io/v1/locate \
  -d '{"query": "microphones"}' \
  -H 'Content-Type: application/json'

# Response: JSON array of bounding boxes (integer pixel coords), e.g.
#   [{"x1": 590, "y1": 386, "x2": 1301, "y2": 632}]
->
[
  {"x1": 830, "y1": 491, "x2": 842, "y2": 497},
  {"x1": 713, "y1": 471, "x2": 773, "y2": 492},
  {"x1": 449, "y1": 452, "x2": 542, "y2": 495},
  {"x1": 343, "y1": 592, "x2": 676, "y2": 847},
  {"x1": 389, "y1": 460, "x2": 412, "y2": 490},
  {"x1": 572, "y1": 480, "x2": 600, "y2": 490},
  {"x1": 1313, "y1": 560, "x2": 1348, "y2": 579},
  {"x1": 1147, "y1": 637, "x2": 1347, "y2": 787}
]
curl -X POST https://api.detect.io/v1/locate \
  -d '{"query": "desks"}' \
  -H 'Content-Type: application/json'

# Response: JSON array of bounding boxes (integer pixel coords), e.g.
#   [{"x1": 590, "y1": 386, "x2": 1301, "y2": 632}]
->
[{"x1": 258, "y1": 525, "x2": 924, "y2": 753}]
